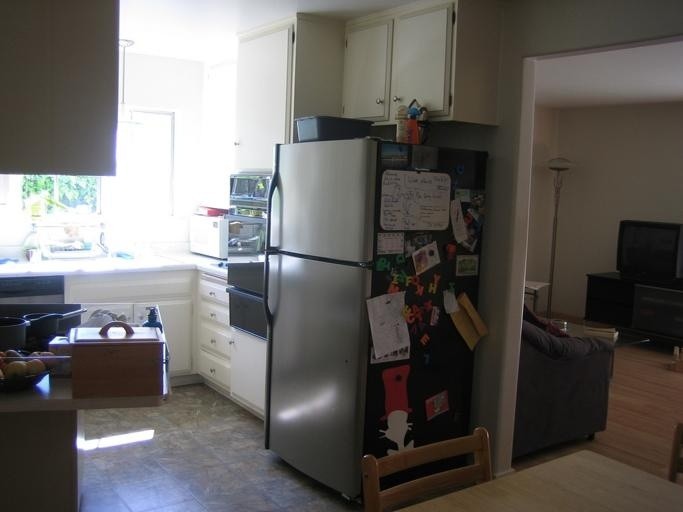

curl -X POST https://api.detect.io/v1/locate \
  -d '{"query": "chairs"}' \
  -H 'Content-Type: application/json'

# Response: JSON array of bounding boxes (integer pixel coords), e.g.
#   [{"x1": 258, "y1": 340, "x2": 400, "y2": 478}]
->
[{"x1": 361, "y1": 427, "x2": 492, "y2": 512}]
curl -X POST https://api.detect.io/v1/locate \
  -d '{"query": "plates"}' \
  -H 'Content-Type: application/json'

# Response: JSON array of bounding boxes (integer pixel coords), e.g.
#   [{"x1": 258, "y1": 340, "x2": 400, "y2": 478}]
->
[{"x1": 0, "y1": 369, "x2": 56, "y2": 392}]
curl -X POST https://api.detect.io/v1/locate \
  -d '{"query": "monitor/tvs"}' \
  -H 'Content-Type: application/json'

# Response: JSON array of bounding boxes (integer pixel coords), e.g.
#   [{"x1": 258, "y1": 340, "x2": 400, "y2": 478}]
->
[{"x1": 616, "y1": 219, "x2": 683, "y2": 290}]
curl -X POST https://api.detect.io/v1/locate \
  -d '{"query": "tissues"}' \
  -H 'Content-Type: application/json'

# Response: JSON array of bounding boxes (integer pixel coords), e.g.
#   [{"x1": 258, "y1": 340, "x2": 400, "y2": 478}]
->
[{"x1": 48, "y1": 336, "x2": 72, "y2": 376}]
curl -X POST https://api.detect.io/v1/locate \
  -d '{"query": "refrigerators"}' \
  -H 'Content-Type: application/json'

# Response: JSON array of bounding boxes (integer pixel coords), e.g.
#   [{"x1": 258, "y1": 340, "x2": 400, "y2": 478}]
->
[{"x1": 261, "y1": 136, "x2": 488, "y2": 512}]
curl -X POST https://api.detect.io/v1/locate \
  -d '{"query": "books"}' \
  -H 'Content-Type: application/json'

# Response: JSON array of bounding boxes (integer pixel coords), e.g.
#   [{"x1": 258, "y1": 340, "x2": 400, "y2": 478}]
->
[{"x1": 586, "y1": 326, "x2": 620, "y2": 340}]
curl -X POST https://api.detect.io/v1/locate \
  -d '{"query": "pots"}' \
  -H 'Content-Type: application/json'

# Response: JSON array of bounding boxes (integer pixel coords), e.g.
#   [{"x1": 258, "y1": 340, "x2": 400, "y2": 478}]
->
[
  {"x1": 22, "y1": 307, "x2": 87, "y2": 338},
  {"x1": 0, "y1": 313, "x2": 64, "y2": 351}
]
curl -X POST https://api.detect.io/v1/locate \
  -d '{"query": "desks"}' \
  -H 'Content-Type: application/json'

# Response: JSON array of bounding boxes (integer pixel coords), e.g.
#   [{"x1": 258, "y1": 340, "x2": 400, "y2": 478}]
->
[
  {"x1": 521, "y1": 282, "x2": 540, "y2": 313},
  {"x1": 387, "y1": 449, "x2": 683, "y2": 512}
]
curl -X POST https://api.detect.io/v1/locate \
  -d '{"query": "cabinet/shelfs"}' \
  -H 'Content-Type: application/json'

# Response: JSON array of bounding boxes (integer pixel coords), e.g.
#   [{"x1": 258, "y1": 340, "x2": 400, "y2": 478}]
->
[
  {"x1": 197, "y1": 272, "x2": 231, "y2": 397},
  {"x1": 243, "y1": 12, "x2": 345, "y2": 144},
  {"x1": 231, "y1": 326, "x2": 267, "y2": 420},
  {"x1": 633, "y1": 281, "x2": 683, "y2": 349},
  {"x1": 342, "y1": 0, "x2": 504, "y2": 127},
  {"x1": 64, "y1": 270, "x2": 194, "y2": 387},
  {"x1": 0, "y1": 411, "x2": 84, "y2": 512},
  {"x1": 581, "y1": 271, "x2": 634, "y2": 329}
]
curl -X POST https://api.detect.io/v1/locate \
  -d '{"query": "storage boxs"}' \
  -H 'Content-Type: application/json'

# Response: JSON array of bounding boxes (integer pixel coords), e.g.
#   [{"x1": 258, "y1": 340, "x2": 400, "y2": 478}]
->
[{"x1": 69, "y1": 322, "x2": 165, "y2": 398}]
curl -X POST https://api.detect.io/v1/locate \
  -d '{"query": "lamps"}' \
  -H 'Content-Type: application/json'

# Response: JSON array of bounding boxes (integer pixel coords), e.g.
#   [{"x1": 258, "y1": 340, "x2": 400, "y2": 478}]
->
[{"x1": 547, "y1": 157, "x2": 572, "y2": 318}]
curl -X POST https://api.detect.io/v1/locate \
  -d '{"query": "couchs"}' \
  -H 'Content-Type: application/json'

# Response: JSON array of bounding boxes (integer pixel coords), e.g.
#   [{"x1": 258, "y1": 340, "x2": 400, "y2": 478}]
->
[{"x1": 512, "y1": 319, "x2": 615, "y2": 458}]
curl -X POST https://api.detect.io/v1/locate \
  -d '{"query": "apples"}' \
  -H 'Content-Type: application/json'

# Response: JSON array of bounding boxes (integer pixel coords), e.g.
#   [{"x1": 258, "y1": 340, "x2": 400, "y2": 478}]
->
[
  {"x1": 6, "y1": 361, "x2": 27, "y2": 378},
  {"x1": 28, "y1": 359, "x2": 45, "y2": 373}
]
